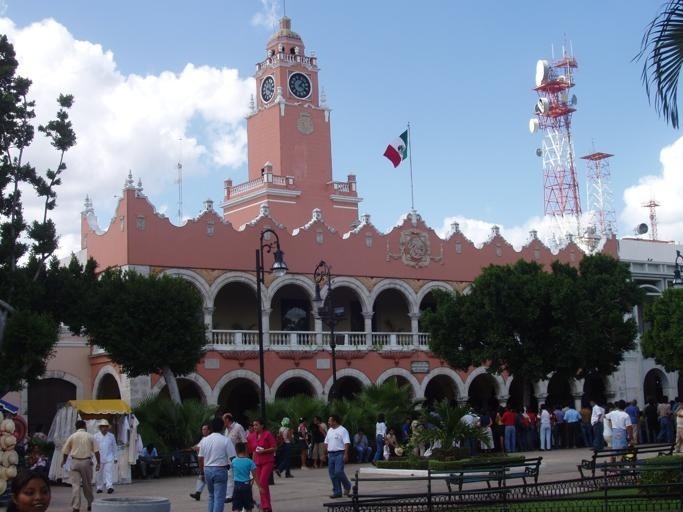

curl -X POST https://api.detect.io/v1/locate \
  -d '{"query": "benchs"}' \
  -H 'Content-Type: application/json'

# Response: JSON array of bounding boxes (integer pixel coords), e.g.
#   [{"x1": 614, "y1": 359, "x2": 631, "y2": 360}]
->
[
  {"x1": 575, "y1": 441, "x2": 678, "y2": 485},
  {"x1": 445, "y1": 455, "x2": 543, "y2": 496}
]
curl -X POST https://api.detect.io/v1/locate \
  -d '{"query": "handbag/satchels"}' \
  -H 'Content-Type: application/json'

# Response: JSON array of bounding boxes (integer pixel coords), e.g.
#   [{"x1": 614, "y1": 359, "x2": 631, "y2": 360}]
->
[{"x1": 298, "y1": 430, "x2": 308, "y2": 437}]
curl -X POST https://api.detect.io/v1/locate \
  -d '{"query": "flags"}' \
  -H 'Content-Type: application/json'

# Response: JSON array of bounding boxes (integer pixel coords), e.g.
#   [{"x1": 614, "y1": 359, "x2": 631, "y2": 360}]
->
[{"x1": 382, "y1": 128, "x2": 408, "y2": 169}]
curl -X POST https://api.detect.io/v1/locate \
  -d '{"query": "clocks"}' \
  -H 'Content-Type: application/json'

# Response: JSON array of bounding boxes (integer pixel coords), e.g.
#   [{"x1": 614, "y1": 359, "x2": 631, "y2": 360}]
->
[
  {"x1": 286, "y1": 70, "x2": 313, "y2": 101},
  {"x1": 258, "y1": 73, "x2": 277, "y2": 104}
]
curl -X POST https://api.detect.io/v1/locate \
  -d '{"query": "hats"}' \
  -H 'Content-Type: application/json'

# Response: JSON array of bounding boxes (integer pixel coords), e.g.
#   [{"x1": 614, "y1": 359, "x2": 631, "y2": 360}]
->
[
  {"x1": 395, "y1": 446, "x2": 404, "y2": 455},
  {"x1": 0, "y1": 413, "x2": 19, "y2": 495},
  {"x1": 97, "y1": 419, "x2": 111, "y2": 429}
]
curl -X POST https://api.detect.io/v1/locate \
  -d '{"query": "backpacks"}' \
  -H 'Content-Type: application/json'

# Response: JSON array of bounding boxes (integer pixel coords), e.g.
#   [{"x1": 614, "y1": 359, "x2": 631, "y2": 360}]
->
[{"x1": 277, "y1": 427, "x2": 290, "y2": 447}]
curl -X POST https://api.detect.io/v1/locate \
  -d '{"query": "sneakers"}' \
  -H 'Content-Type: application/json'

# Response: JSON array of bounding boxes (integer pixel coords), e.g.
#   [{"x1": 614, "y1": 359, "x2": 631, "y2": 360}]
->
[
  {"x1": 73, "y1": 509, "x2": 79, "y2": 511},
  {"x1": 107, "y1": 487, "x2": 114, "y2": 494},
  {"x1": 96, "y1": 490, "x2": 103, "y2": 493},
  {"x1": 501, "y1": 444, "x2": 591, "y2": 453},
  {"x1": 302, "y1": 467, "x2": 310, "y2": 470},
  {"x1": 87, "y1": 507, "x2": 91, "y2": 512}
]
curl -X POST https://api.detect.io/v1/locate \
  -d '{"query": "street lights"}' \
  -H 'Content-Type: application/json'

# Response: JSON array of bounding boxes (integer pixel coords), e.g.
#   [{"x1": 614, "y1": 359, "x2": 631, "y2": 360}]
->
[
  {"x1": 670, "y1": 248, "x2": 682, "y2": 286},
  {"x1": 311, "y1": 258, "x2": 339, "y2": 399},
  {"x1": 253, "y1": 228, "x2": 289, "y2": 426}
]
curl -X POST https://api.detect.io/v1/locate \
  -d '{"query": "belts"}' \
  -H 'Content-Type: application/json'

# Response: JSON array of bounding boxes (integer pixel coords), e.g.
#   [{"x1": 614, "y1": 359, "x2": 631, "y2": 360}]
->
[{"x1": 72, "y1": 457, "x2": 91, "y2": 460}]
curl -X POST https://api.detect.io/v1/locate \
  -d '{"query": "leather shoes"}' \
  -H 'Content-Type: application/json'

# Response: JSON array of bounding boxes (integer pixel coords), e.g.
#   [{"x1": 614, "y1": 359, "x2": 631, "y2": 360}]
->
[
  {"x1": 344, "y1": 488, "x2": 350, "y2": 495},
  {"x1": 274, "y1": 469, "x2": 282, "y2": 477},
  {"x1": 189, "y1": 491, "x2": 202, "y2": 502},
  {"x1": 224, "y1": 498, "x2": 234, "y2": 503},
  {"x1": 285, "y1": 473, "x2": 295, "y2": 478},
  {"x1": 329, "y1": 494, "x2": 343, "y2": 499}
]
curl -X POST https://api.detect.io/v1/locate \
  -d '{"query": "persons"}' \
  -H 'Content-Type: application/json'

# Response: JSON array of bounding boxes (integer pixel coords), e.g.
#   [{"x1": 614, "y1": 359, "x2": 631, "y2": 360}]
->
[
  {"x1": 3, "y1": 469, "x2": 51, "y2": 511},
  {"x1": 91, "y1": 418, "x2": 118, "y2": 494},
  {"x1": 353, "y1": 406, "x2": 517, "y2": 466},
  {"x1": 59, "y1": 419, "x2": 101, "y2": 511},
  {"x1": 179, "y1": 413, "x2": 352, "y2": 503},
  {"x1": 245, "y1": 416, "x2": 277, "y2": 511},
  {"x1": 138, "y1": 442, "x2": 161, "y2": 479},
  {"x1": 197, "y1": 416, "x2": 237, "y2": 511},
  {"x1": 230, "y1": 441, "x2": 256, "y2": 512},
  {"x1": 517, "y1": 395, "x2": 683, "y2": 475}
]
